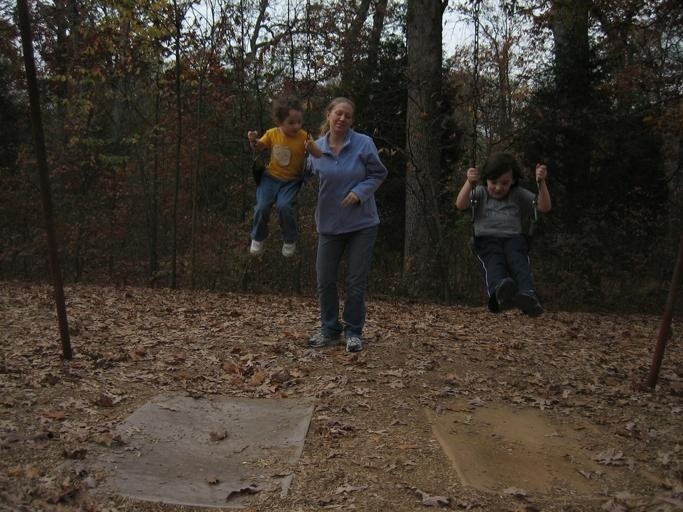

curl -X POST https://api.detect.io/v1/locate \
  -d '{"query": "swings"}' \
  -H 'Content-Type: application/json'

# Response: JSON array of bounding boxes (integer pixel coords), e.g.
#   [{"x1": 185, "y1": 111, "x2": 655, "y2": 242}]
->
[
  {"x1": 222, "y1": 0, "x2": 311, "y2": 193},
  {"x1": 468, "y1": 2, "x2": 561, "y2": 254}
]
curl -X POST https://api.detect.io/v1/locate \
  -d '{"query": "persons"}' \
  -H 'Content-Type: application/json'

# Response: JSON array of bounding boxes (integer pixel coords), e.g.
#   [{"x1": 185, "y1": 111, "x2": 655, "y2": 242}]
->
[
  {"x1": 304, "y1": 95, "x2": 391, "y2": 353},
  {"x1": 451, "y1": 150, "x2": 555, "y2": 319},
  {"x1": 245, "y1": 93, "x2": 322, "y2": 257}
]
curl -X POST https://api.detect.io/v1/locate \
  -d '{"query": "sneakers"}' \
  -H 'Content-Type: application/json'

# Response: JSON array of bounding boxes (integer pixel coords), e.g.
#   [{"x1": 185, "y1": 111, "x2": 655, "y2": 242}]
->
[
  {"x1": 309, "y1": 333, "x2": 342, "y2": 348},
  {"x1": 345, "y1": 336, "x2": 364, "y2": 352},
  {"x1": 515, "y1": 292, "x2": 544, "y2": 318},
  {"x1": 250, "y1": 239, "x2": 264, "y2": 255},
  {"x1": 496, "y1": 277, "x2": 517, "y2": 312},
  {"x1": 282, "y1": 241, "x2": 296, "y2": 258}
]
